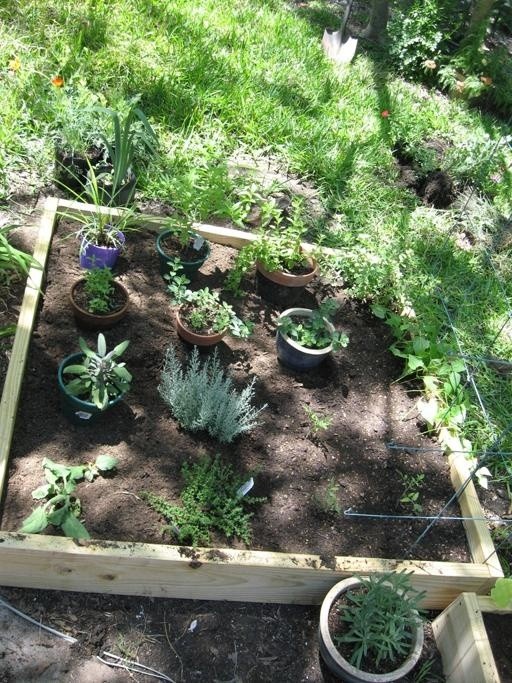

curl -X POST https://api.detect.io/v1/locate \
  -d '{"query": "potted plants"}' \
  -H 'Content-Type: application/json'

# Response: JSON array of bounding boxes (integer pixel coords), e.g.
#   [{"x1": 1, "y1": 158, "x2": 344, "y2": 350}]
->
[
  {"x1": 70, "y1": 255, "x2": 132, "y2": 333},
  {"x1": 46, "y1": 67, "x2": 142, "y2": 193},
  {"x1": 275, "y1": 298, "x2": 351, "y2": 373},
  {"x1": 163, "y1": 257, "x2": 254, "y2": 347},
  {"x1": 156, "y1": 205, "x2": 211, "y2": 284},
  {"x1": 58, "y1": 332, "x2": 135, "y2": 427},
  {"x1": 87, "y1": 122, "x2": 162, "y2": 209},
  {"x1": 45, "y1": 141, "x2": 155, "y2": 272},
  {"x1": 224, "y1": 193, "x2": 320, "y2": 307},
  {"x1": 319, "y1": 568, "x2": 426, "y2": 683}
]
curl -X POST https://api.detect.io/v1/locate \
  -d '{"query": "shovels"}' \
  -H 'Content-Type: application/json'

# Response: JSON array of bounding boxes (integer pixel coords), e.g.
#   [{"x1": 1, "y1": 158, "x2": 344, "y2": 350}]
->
[{"x1": 323, "y1": 0, "x2": 358, "y2": 66}]
